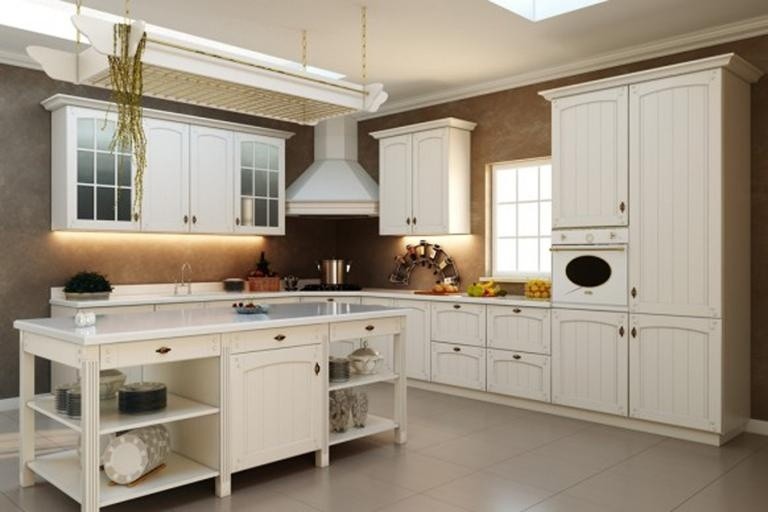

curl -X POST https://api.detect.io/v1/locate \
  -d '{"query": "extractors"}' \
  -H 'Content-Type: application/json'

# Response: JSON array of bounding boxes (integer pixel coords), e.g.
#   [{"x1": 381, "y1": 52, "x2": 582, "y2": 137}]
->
[{"x1": 286, "y1": 115, "x2": 379, "y2": 216}]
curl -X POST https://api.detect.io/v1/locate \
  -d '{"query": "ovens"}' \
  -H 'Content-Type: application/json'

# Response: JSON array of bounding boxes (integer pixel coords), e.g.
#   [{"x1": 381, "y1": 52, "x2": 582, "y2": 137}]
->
[{"x1": 545, "y1": 241, "x2": 633, "y2": 311}]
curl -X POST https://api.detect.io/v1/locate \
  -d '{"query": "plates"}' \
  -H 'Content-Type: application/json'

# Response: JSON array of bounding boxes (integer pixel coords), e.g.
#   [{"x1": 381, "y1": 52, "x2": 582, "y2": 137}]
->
[
  {"x1": 330, "y1": 358, "x2": 350, "y2": 383},
  {"x1": 234, "y1": 304, "x2": 270, "y2": 314},
  {"x1": 118, "y1": 383, "x2": 167, "y2": 413},
  {"x1": 67, "y1": 389, "x2": 81, "y2": 416},
  {"x1": 56, "y1": 387, "x2": 66, "y2": 411},
  {"x1": 103, "y1": 424, "x2": 170, "y2": 484}
]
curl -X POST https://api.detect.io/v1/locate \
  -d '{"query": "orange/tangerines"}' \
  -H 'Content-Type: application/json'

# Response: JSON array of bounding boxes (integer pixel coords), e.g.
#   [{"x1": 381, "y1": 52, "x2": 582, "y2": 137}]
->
[{"x1": 524, "y1": 279, "x2": 552, "y2": 299}]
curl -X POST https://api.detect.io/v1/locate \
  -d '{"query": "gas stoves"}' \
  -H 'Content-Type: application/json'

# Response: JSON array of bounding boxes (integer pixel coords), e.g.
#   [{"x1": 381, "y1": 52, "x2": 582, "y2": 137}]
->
[{"x1": 300, "y1": 284, "x2": 361, "y2": 292}]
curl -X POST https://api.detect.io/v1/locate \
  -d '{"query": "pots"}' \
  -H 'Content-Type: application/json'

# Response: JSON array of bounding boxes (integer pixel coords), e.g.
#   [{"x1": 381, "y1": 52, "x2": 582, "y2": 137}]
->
[{"x1": 314, "y1": 256, "x2": 351, "y2": 285}]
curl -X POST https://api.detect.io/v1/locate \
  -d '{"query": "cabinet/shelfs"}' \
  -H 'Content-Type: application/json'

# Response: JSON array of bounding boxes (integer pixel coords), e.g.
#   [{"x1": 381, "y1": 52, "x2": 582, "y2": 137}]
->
[
  {"x1": 430, "y1": 342, "x2": 487, "y2": 394},
  {"x1": 550, "y1": 305, "x2": 750, "y2": 434},
  {"x1": 550, "y1": 227, "x2": 628, "y2": 311},
  {"x1": 300, "y1": 288, "x2": 360, "y2": 304},
  {"x1": 430, "y1": 303, "x2": 487, "y2": 350},
  {"x1": 14, "y1": 302, "x2": 414, "y2": 512},
  {"x1": 370, "y1": 117, "x2": 477, "y2": 237},
  {"x1": 155, "y1": 291, "x2": 301, "y2": 314},
  {"x1": 361, "y1": 296, "x2": 431, "y2": 384},
  {"x1": 233, "y1": 129, "x2": 286, "y2": 236},
  {"x1": 50, "y1": 293, "x2": 155, "y2": 397},
  {"x1": 139, "y1": 119, "x2": 233, "y2": 234},
  {"x1": 627, "y1": 65, "x2": 762, "y2": 309},
  {"x1": 551, "y1": 85, "x2": 628, "y2": 228},
  {"x1": 486, "y1": 304, "x2": 553, "y2": 355},
  {"x1": 50, "y1": 106, "x2": 140, "y2": 234},
  {"x1": 485, "y1": 348, "x2": 551, "y2": 403}
]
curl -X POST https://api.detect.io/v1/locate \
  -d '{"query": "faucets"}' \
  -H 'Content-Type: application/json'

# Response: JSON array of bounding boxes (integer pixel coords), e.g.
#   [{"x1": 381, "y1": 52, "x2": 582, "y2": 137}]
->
[{"x1": 174, "y1": 262, "x2": 193, "y2": 295}]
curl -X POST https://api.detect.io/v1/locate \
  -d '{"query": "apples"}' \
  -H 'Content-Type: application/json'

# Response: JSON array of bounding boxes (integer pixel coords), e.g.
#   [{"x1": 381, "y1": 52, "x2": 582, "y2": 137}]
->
[{"x1": 469, "y1": 285, "x2": 483, "y2": 297}]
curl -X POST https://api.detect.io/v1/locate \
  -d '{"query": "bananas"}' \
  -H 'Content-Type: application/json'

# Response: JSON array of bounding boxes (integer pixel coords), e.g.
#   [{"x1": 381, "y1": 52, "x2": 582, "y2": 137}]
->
[{"x1": 478, "y1": 281, "x2": 500, "y2": 295}]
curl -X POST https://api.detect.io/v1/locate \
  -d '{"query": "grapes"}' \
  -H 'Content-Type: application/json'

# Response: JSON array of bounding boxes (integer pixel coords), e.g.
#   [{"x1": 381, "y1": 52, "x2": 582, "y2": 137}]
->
[{"x1": 233, "y1": 302, "x2": 261, "y2": 309}]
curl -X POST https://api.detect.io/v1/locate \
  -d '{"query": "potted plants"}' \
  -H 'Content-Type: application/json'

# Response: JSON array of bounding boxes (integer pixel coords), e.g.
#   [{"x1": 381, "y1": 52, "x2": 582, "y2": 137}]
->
[{"x1": 62, "y1": 271, "x2": 112, "y2": 300}]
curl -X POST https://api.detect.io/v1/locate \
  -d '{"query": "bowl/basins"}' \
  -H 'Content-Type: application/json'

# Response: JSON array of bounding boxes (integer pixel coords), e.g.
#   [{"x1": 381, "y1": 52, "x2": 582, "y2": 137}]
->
[
  {"x1": 349, "y1": 342, "x2": 383, "y2": 375},
  {"x1": 100, "y1": 369, "x2": 126, "y2": 399}
]
